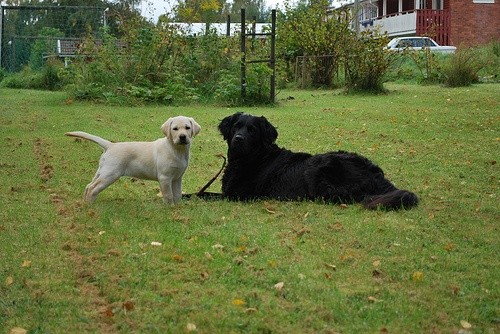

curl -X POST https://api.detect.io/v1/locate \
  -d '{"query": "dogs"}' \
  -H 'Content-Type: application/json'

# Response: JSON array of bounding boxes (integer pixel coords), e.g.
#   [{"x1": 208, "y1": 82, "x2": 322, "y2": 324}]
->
[
  {"x1": 182, "y1": 111, "x2": 418, "y2": 213},
  {"x1": 63, "y1": 116, "x2": 202, "y2": 208}
]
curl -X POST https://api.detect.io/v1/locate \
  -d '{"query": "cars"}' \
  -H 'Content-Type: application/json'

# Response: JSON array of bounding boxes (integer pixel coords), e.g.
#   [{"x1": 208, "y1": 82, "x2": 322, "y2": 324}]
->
[{"x1": 367, "y1": 36, "x2": 458, "y2": 57}]
